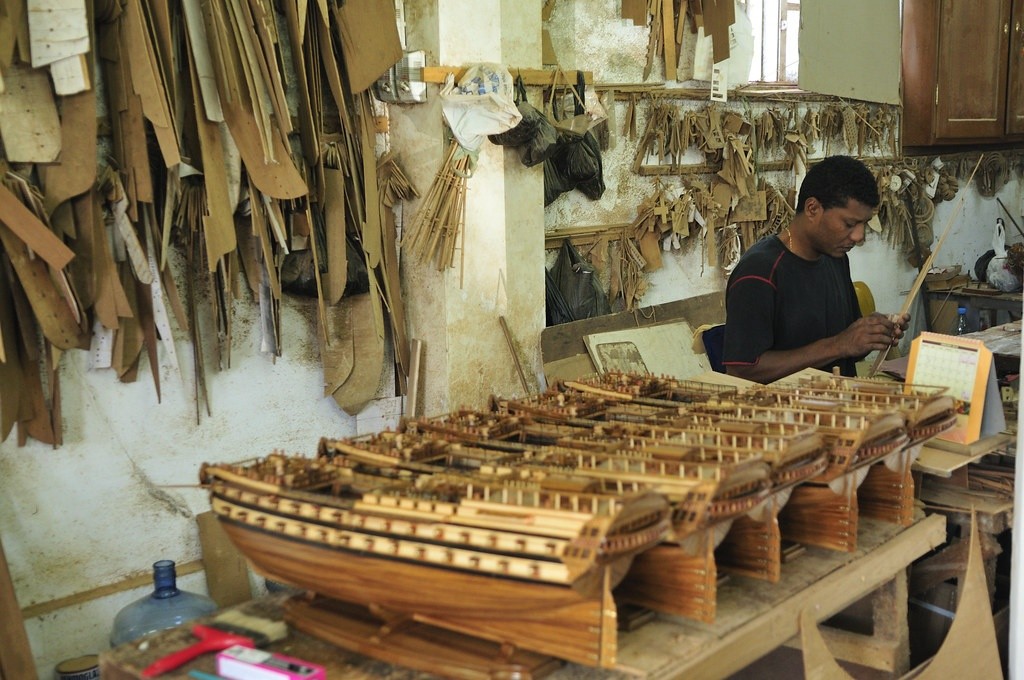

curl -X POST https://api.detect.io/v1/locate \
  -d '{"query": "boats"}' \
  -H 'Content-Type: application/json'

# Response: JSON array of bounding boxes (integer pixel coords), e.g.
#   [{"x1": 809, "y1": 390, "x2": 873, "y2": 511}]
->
[{"x1": 198, "y1": 369, "x2": 970, "y2": 670}]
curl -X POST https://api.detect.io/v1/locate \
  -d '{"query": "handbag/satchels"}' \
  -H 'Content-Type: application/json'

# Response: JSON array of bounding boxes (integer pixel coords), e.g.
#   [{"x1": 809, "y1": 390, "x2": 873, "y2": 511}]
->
[
  {"x1": 551, "y1": 238, "x2": 611, "y2": 320},
  {"x1": 974, "y1": 218, "x2": 1013, "y2": 281},
  {"x1": 987, "y1": 223, "x2": 1021, "y2": 292},
  {"x1": 518, "y1": 104, "x2": 556, "y2": 165},
  {"x1": 545, "y1": 268, "x2": 573, "y2": 326},
  {"x1": 543, "y1": 66, "x2": 606, "y2": 204},
  {"x1": 487, "y1": 76, "x2": 542, "y2": 147}
]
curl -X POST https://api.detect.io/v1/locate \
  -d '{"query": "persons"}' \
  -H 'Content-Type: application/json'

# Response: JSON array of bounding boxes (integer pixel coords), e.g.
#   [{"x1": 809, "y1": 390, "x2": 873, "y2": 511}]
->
[{"x1": 721, "y1": 155, "x2": 913, "y2": 387}]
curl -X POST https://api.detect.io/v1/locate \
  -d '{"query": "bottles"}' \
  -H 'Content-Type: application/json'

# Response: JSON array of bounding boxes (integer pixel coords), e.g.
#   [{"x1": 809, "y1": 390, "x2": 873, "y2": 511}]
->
[
  {"x1": 110, "y1": 560, "x2": 218, "y2": 648},
  {"x1": 950, "y1": 308, "x2": 971, "y2": 336}
]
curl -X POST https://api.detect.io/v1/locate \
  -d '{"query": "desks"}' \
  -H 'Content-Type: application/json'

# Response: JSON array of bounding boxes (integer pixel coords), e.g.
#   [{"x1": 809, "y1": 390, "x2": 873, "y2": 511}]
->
[{"x1": 98, "y1": 512, "x2": 946, "y2": 680}]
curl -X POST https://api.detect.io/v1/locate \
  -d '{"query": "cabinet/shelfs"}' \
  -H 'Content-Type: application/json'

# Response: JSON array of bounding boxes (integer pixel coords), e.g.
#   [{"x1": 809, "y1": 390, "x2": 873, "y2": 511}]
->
[{"x1": 898, "y1": 0, "x2": 1024, "y2": 157}]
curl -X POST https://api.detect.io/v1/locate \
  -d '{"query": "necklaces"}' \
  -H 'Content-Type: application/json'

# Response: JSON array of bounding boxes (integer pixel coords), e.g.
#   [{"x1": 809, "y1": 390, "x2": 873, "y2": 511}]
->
[{"x1": 784, "y1": 227, "x2": 794, "y2": 252}]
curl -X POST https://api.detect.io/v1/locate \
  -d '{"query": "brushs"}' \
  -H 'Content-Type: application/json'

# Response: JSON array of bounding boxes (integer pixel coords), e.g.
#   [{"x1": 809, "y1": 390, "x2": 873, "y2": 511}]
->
[{"x1": 137, "y1": 599, "x2": 292, "y2": 678}]
